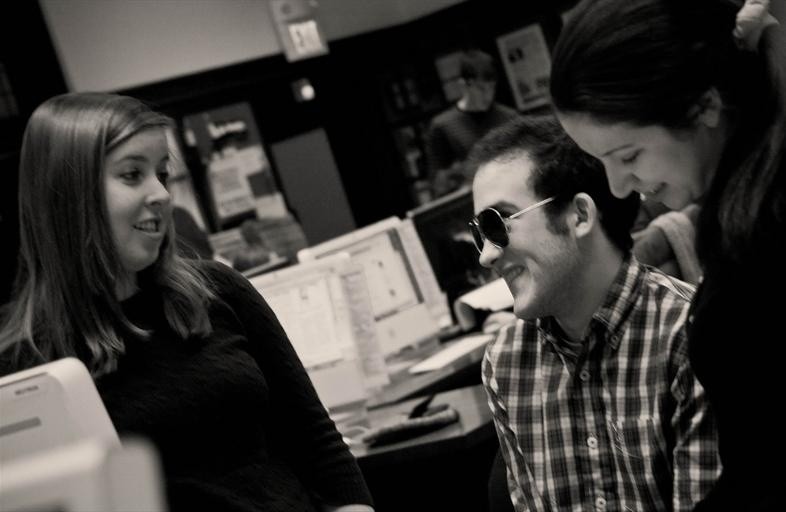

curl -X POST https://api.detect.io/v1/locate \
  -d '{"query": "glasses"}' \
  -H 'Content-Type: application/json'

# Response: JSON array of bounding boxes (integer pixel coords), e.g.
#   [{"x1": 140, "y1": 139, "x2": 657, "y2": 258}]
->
[{"x1": 467, "y1": 197, "x2": 555, "y2": 254}]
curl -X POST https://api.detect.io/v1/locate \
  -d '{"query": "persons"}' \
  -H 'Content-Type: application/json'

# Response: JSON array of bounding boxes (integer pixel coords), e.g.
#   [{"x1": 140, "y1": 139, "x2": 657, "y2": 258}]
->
[
  {"x1": 548, "y1": 0, "x2": 786, "y2": 512},
  {"x1": 428, "y1": 51, "x2": 522, "y2": 199},
  {"x1": 0, "y1": 93, "x2": 374, "y2": 512},
  {"x1": 468, "y1": 114, "x2": 724, "y2": 512},
  {"x1": 172, "y1": 204, "x2": 237, "y2": 274}
]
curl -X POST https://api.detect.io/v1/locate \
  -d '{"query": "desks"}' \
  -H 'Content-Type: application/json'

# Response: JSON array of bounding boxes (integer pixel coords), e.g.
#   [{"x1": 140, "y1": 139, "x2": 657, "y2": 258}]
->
[
  {"x1": 366, "y1": 331, "x2": 497, "y2": 410},
  {"x1": 350, "y1": 384, "x2": 500, "y2": 496}
]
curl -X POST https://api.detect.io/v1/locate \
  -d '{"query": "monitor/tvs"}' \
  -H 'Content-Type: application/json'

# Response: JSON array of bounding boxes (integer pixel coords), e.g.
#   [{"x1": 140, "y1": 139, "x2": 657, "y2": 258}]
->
[
  {"x1": 246, "y1": 250, "x2": 391, "y2": 435},
  {"x1": 297, "y1": 216, "x2": 453, "y2": 363},
  {"x1": 1, "y1": 352, "x2": 130, "y2": 471},
  {"x1": 406, "y1": 185, "x2": 502, "y2": 342}
]
